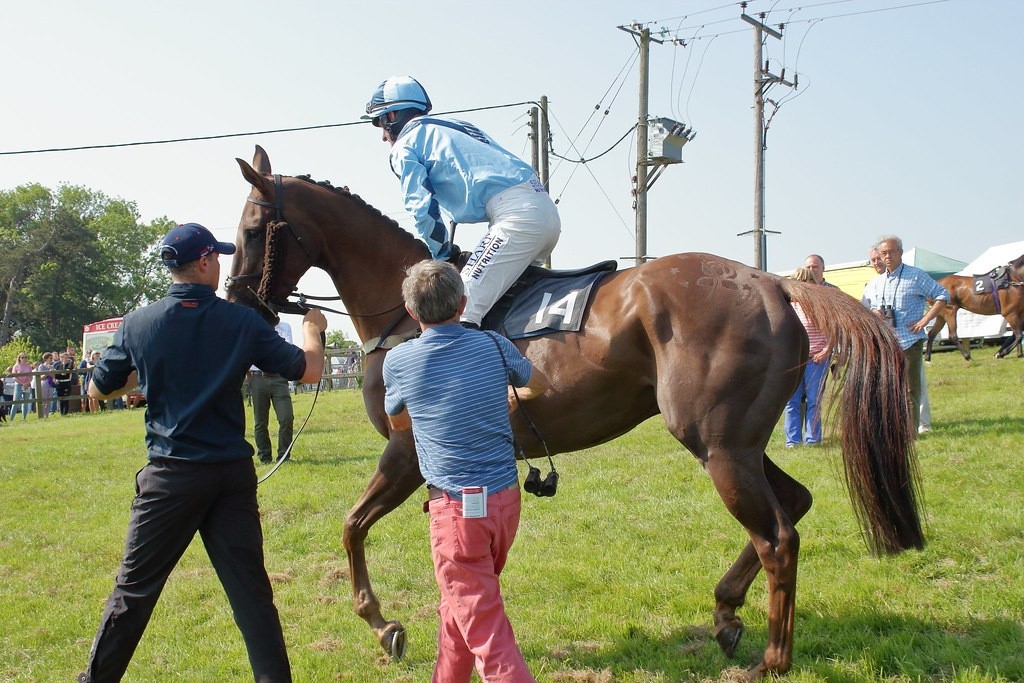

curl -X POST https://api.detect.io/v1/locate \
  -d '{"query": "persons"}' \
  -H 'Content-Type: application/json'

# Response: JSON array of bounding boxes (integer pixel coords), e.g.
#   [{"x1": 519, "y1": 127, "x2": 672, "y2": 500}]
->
[
  {"x1": 0, "y1": 345, "x2": 142, "y2": 423},
  {"x1": 78, "y1": 223, "x2": 328, "y2": 683},
  {"x1": 382, "y1": 259, "x2": 548, "y2": 683},
  {"x1": 361, "y1": 73, "x2": 561, "y2": 343},
  {"x1": 250, "y1": 322, "x2": 295, "y2": 466},
  {"x1": 784, "y1": 254, "x2": 841, "y2": 449},
  {"x1": 861, "y1": 234, "x2": 949, "y2": 434}
]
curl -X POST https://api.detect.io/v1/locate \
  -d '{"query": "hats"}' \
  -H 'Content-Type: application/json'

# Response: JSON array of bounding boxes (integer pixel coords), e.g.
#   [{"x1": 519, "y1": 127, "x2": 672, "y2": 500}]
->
[{"x1": 161, "y1": 222, "x2": 236, "y2": 266}]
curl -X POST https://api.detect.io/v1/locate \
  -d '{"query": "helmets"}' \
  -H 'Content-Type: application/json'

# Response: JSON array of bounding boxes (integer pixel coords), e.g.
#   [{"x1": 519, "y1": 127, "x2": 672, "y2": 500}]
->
[{"x1": 360, "y1": 76, "x2": 432, "y2": 117}]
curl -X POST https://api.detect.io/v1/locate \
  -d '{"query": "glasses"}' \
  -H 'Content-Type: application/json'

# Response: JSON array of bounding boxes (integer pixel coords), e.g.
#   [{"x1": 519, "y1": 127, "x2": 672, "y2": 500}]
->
[
  {"x1": 20, "y1": 357, "x2": 27, "y2": 358},
  {"x1": 50, "y1": 358, "x2": 53, "y2": 359},
  {"x1": 96, "y1": 353, "x2": 100, "y2": 354}
]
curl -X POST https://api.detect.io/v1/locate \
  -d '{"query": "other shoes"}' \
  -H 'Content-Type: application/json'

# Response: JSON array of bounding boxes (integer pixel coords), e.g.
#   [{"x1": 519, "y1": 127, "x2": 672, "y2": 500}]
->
[
  {"x1": 78, "y1": 672, "x2": 87, "y2": 683},
  {"x1": 918, "y1": 425, "x2": 933, "y2": 434}
]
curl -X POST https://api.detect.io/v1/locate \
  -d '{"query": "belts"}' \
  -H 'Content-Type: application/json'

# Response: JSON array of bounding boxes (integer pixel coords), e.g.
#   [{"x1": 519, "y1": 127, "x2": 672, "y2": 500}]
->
[{"x1": 251, "y1": 371, "x2": 278, "y2": 377}]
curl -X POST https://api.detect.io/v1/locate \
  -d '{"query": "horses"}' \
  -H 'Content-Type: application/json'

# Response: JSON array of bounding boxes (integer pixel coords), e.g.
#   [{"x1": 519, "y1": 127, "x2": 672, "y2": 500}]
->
[
  {"x1": 225, "y1": 145, "x2": 933, "y2": 683},
  {"x1": 924, "y1": 253, "x2": 1024, "y2": 359}
]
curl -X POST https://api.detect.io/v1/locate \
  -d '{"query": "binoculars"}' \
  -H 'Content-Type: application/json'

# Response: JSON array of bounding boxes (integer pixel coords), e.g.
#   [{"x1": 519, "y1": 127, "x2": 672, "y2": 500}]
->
[
  {"x1": 524, "y1": 467, "x2": 560, "y2": 497},
  {"x1": 879, "y1": 304, "x2": 896, "y2": 328}
]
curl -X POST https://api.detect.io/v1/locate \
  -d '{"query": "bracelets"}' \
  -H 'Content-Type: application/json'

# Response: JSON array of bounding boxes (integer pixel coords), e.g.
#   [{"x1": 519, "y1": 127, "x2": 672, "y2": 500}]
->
[{"x1": 823, "y1": 348, "x2": 827, "y2": 352}]
